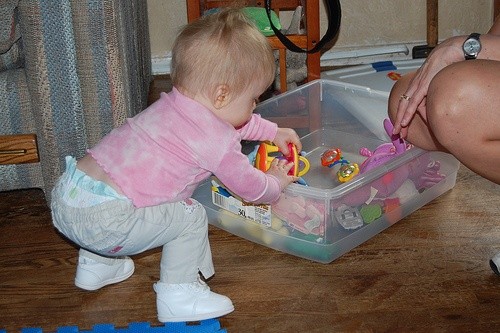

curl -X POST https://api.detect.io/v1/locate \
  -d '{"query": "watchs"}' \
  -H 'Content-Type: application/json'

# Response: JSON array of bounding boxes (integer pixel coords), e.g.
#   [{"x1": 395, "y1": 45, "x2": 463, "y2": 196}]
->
[{"x1": 462, "y1": 32, "x2": 481, "y2": 60}]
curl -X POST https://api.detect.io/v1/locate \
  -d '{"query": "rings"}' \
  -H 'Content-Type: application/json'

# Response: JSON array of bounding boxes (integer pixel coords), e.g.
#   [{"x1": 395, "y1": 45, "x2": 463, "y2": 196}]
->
[{"x1": 401, "y1": 94, "x2": 410, "y2": 102}]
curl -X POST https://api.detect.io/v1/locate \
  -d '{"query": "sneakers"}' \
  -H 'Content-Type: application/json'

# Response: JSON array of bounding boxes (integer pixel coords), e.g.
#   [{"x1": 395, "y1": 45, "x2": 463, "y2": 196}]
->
[
  {"x1": 153, "y1": 279, "x2": 236, "y2": 324},
  {"x1": 74, "y1": 249, "x2": 135, "y2": 292}
]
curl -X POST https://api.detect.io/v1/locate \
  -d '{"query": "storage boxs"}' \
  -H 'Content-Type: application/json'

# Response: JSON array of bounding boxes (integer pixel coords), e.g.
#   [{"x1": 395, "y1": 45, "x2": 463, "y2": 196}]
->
[{"x1": 192, "y1": 56, "x2": 459, "y2": 264}]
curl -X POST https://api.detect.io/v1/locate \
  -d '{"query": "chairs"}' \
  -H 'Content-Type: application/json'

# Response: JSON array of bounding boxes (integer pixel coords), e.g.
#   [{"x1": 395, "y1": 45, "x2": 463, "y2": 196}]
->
[{"x1": 187, "y1": 0, "x2": 322, "y2": 150}]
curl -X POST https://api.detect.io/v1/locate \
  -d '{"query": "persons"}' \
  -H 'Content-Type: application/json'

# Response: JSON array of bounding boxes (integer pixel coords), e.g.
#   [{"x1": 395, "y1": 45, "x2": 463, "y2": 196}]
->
[
  {"x1": 388, "y1": 16, "x2": 500, "y2": 274},
  {"x1": 51, "y1": 8, "x2": 303, "y2": 322}
]
